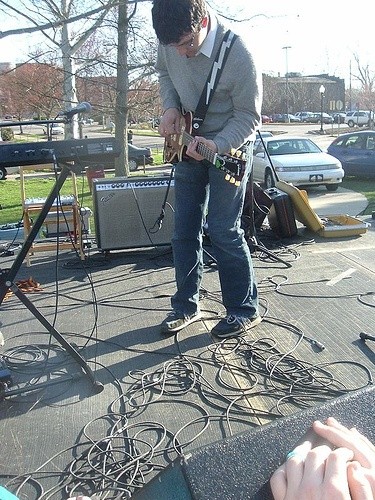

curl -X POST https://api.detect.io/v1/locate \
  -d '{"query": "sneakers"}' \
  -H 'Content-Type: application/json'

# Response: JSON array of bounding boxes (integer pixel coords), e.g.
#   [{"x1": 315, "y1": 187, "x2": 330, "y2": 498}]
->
[
  {"x1": 211, "y1": 309, "x2": 262, "y2": 337},
  {"x1": 161, "y1": 308, "x2": 202, "y2": 333}
]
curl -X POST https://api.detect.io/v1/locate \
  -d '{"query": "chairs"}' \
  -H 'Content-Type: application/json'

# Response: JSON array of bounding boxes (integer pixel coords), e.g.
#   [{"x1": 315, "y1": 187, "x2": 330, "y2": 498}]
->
[{"x1": 19, "y1": 160, "x2": 85, "y2": 267}]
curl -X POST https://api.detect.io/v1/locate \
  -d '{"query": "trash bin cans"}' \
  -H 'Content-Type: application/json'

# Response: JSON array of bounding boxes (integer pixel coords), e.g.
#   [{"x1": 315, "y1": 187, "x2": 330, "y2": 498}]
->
[{"x1": 85, "y1": 164, "x2": 105, "y2": 193}]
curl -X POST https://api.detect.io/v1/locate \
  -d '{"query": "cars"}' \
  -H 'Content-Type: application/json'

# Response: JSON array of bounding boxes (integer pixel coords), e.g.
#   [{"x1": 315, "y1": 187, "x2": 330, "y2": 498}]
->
[
  {"x1": 260, "y1": 114, "x2": 273, "y2": 124},
  {"x1": 308, "y1": 113, "x2": 334, "y2": 124},
  {"x1": 328, "y1": 111, "x2": 347, "y2": 124},
  {"x1": 269, "y1": 111, "x2": 315, "y2": 123},
  {"x1": 68, "y1": 136, "x2": 153, "y2": 171},
  {"x1": 253, "y1": 137, "x2": 345, "y2": 193},
  {"x1": 43, "y1": 123, "x2": 64, "y2": 135},
  {"x1": 0, "y1": 166, "x2": 21, "y2": 179},
  {"x1": 326, "y1": 131, "x2": 375, "y2": 178}
]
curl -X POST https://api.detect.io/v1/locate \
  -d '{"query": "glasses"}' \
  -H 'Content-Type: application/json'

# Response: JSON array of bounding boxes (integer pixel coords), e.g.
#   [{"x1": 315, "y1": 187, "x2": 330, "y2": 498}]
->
[{"x1": 162, "y1": 28, "x2": 198, "y2": 49}]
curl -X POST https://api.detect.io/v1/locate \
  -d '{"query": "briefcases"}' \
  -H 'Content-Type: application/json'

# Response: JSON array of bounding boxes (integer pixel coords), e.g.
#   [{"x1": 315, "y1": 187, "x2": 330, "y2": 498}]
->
[
  {"x1": 265, "y1": 186, "x2": 297, "y2": 237},
  {"x1": 276, "y1": 180, "x2": 372, "y2": 237}
]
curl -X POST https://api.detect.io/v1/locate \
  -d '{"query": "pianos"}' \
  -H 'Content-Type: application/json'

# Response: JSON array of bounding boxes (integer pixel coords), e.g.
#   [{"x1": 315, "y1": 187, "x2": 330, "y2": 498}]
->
[{"x1": 0, "y1": 137, "x2": 122, "y2": 393}]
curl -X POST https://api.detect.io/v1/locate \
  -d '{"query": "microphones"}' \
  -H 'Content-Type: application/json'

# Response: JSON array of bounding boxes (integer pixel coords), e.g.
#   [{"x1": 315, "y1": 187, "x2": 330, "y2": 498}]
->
[{"x1": 59, "y1": 102, "x2": 91, "y2": 117}]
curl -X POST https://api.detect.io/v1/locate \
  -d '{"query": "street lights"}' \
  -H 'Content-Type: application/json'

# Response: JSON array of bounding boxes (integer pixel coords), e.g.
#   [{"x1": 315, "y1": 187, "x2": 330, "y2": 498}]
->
[
  {"x1": 318, "y1": 84, "x2": 327, "y2": 135},
  {"x1": 282, "y1": 46, "x2": 292, "y2": 123}
]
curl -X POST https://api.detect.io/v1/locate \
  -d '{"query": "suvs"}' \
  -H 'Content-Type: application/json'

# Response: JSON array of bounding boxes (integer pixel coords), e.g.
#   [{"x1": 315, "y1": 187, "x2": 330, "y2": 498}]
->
[{"x1": 344, "y1": 110, "x2": 375, "y2": 128}]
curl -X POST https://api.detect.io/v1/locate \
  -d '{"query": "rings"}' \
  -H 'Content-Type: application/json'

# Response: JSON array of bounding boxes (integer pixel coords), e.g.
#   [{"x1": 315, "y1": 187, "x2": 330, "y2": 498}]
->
[
  {"x1": 164, "y1": 129, "x2": 168, "y2": 134},
  {"x1": 286, "y1": 450, "x2": 306, "y2": 463}
]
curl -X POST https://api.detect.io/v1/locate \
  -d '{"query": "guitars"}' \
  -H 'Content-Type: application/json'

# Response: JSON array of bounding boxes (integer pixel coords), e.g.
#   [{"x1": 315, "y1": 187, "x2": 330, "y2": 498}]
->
[{"x1": 163, "y1": 104, "x2": 248, "y2": 186}]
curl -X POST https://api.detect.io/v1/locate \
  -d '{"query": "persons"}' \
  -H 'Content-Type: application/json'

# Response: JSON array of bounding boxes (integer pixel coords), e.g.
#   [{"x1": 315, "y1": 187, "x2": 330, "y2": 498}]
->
[
  {"x1": 127, "y1": 130, "x2": 133, "y2": 145},
  {"x1": 109, "y1": 121, "x2": 115, "y2": 135},
  {"x1": 269, "y1": 417, "x2": 375, "y2": 500},
  {"x1": 149, "y1": 0, "x2": 264, "y2": 339}
]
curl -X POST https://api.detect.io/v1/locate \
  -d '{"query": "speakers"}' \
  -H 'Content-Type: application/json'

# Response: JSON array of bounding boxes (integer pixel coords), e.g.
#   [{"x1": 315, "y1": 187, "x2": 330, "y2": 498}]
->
[
  {"x1": 90, "y1": 176, "x2": 175, "y2": 251},
  {"x1": 134, "y1": 386, "x2": 375, "y2": 500}
]
